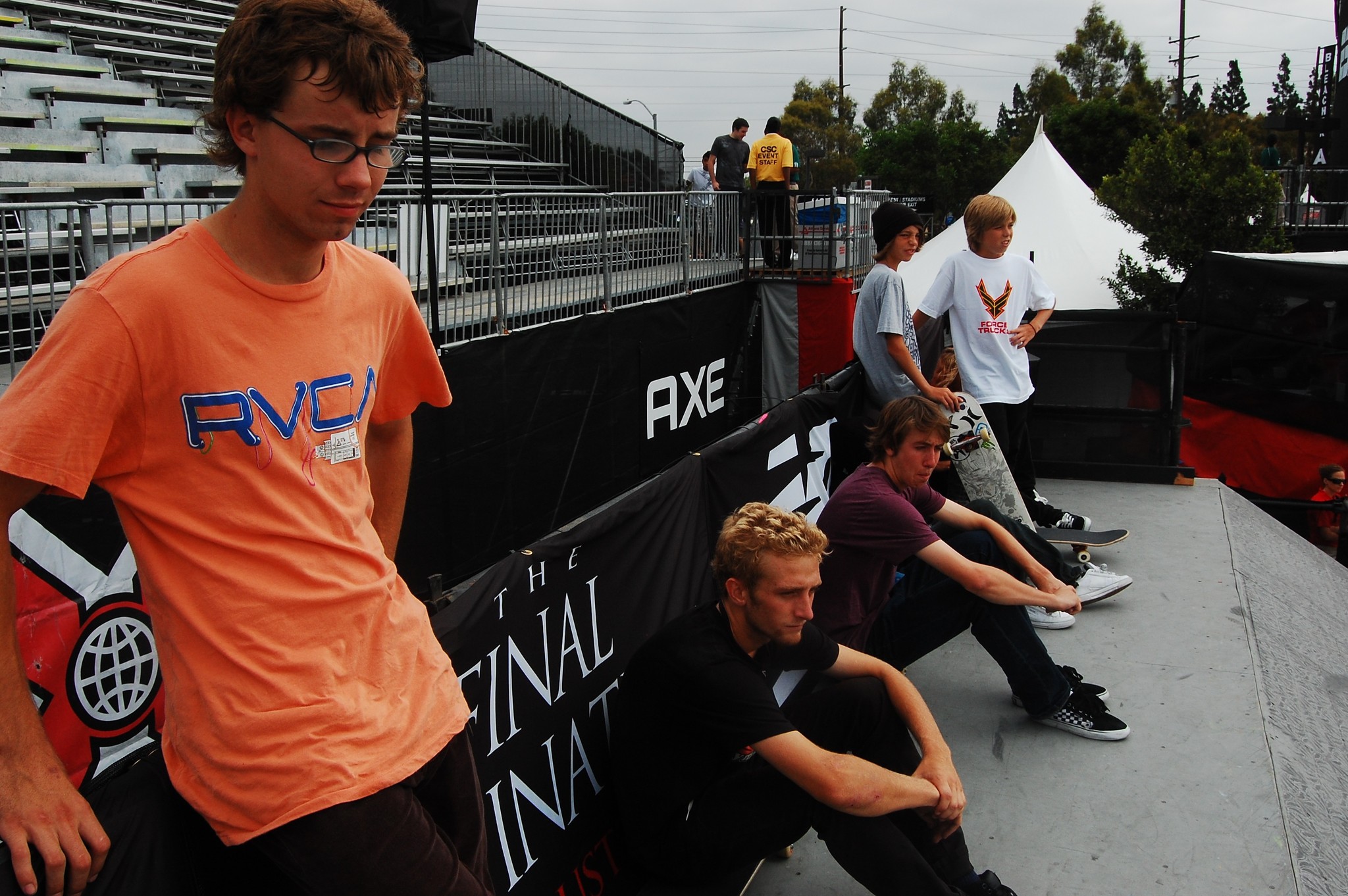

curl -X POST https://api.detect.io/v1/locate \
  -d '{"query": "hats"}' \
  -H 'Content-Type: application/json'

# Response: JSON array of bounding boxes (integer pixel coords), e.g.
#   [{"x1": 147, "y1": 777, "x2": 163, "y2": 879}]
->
[
  {"x1": 703, "y1": 151, "x2": 711, "y2": 157},
  {"x1": 766, "y1": 117, "x2": 780, "y2": 130},
  {"x1": 872, "y1": 201, "x2": 924, "y2": 252}
]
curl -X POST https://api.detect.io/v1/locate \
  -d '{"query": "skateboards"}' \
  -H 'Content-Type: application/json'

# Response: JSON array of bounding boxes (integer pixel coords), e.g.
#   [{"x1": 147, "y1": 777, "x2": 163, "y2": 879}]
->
[
  {"x1": 1032, "y1": 528, "x2": 1130, "y2": 562},
  {"x1": 930, "y1": 391, "x2": 1037, "y2": 538},
  {"x1": 729, "y1": 841, "x2": 794, "y2": 896}
]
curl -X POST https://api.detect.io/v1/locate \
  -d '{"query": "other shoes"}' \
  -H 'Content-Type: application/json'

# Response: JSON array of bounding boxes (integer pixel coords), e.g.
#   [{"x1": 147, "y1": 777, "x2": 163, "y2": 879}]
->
[
  {"x1": 777, "y1": 255, "x2": 792, "y2": 269},
  {"x1": 957, "y1": 869, "x2": 1017, "y2": 896},
  {"x1": 769, "y1": 262, "x2": 777, "y2": 268}
]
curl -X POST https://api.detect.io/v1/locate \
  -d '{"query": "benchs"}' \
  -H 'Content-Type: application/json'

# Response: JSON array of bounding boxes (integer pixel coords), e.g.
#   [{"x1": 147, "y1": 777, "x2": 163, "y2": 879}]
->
[{"x1": 0, "y1": 0, "x2": 680, "y2": 352}]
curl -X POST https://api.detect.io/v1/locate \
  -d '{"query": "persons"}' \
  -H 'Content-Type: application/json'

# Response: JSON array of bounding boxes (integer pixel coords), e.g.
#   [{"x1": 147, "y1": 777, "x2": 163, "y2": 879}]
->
[
  {"x1": 687, "y1": 151, "x2": 717, "y2": 259},
  {"x1": 811, "y1": 396, "x2": 1131, "y2": 742},
  {"x1": 709, "y1": 118, "x2": 749, "y2": 264},
  {"x1": 747, "y1": 117, "x2": 800, "y2": 271},
  {"x1": 854, "y1": 202, "x2": 969, "y2": 501},
  {"x1": 914, "y1": 194, "x2": 1091, "y2": 533},
  {"x1": 0, "y1": 0, "x2": 484, "y2": 896},
  {"x1": 596, "y1": 501, "x2": 1016, "y2": 896}
]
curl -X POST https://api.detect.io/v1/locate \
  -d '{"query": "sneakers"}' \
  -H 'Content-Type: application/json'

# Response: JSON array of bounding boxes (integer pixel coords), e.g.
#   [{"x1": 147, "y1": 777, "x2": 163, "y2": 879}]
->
[
  {"x1": 1033, "y1": 489, "x2": 1048, "y2": 505},
  {"x1": 1025, "y1": 605, "x2": 1076, "y2": 629},
  {"x1": 1075, "y1": 568, "x2": 1133, "y2": 606},
  {"x1": 1011, "y1": 665, "x2": 1109, "y2": 707},
  {"x1": 1050, "y1": 511, "x2": 1091, "y2": 531},
  {"x1": 1030, "y1": 688, "x2": 1131, "y2": 741}
]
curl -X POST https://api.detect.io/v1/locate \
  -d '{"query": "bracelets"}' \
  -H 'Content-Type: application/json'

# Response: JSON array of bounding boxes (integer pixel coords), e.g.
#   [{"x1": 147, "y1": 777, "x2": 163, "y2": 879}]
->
[{"x1": 1028, "y1": 322, "x2": 1037, "y2": 333}]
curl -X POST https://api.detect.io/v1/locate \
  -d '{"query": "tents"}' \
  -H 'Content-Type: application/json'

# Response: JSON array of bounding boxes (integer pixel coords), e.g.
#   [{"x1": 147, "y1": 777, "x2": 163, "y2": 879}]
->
[{"x1": 897, "y1": 114, "x2": 1187, "y2": 316}]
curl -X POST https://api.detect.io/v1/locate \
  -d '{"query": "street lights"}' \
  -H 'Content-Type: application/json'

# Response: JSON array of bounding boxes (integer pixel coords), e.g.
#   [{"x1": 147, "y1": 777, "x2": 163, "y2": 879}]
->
[{"x1": 623, "y1": 99, "x2": 659, "y2": 198}]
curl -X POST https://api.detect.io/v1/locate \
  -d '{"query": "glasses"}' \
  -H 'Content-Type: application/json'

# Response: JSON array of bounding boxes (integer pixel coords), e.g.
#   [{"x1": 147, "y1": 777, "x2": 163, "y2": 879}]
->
[{"x1": 267, "y1": 116, "x2": 409, "y2": 168}]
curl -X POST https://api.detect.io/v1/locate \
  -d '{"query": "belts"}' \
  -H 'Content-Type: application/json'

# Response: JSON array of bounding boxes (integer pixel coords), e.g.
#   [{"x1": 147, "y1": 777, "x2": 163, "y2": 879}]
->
[{"x1": 791, "y1": 181, "x2": 798, "y2": 184}]
